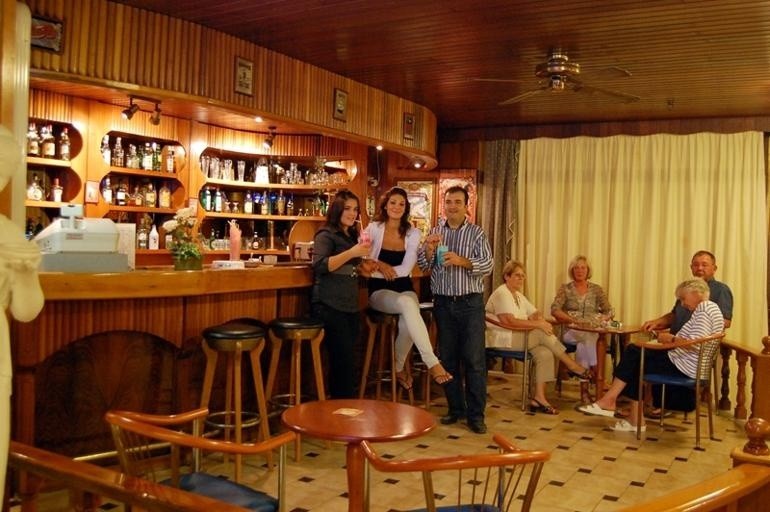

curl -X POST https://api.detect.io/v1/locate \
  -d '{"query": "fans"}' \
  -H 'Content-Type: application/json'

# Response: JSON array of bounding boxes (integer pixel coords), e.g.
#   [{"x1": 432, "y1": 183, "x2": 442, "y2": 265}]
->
[{"x1": 474, "y1": 54, "x2": 641, "y2": 105}]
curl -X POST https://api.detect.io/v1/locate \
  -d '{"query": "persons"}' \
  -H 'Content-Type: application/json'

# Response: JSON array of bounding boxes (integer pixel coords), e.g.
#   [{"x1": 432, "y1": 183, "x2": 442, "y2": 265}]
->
[
  {"x1": 577, "y1": 277, "x2": 724, "y2": 433},
  {"x1": 550, "y1": 256, "x2": 612, "y2": 404},
  {"x1": 614, "y1": 251, "x2": 733, "y2": 419},
  {"x1": 485, "y1": 261, "x2": 595, "y2": 415},
  {"x1": 358, "y1": 187, "x2": 454, "y2": 391},
  {"x1": 417, "y1": 186, "x2": 494, "y2": 433},
  {"x1": 0, "y1": 126, "x2": 44, "y2": 511},
  {"x1": 311, "y1": 191, "x2": 379, "y2": 400}
]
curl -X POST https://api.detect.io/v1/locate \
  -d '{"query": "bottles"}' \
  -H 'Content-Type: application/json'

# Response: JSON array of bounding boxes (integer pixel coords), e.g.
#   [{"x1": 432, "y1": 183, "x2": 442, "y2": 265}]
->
[
  {"x1": 101, "y1": 135, "x2": 176, "y2": 252},
  {"x1": 200, "y1": 186, "x2": 328, "y2": 251},
  {"x1": 25, "y1": 121, "x2": 71, "y2": 241}
]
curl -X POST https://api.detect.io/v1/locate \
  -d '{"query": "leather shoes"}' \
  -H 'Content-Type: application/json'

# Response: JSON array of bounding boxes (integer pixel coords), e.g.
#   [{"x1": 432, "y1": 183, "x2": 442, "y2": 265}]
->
[
  {"x1": 441, "y1": 414, "x2": 459, "y2": 424},
  {"x1": 468, "y1": 417, "x2": 486, "y2": 433}
]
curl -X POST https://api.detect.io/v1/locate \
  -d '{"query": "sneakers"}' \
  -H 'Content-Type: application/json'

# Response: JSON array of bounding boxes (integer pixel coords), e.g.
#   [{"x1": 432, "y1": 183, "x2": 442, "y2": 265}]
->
[{"x1": 580, "y1": 381, "x2": 594, "y2": 403}]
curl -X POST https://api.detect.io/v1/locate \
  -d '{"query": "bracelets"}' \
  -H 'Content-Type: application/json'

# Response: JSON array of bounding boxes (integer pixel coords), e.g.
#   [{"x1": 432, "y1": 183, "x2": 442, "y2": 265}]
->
[{"x1": 671, "y1": 337, "x2": 674, "y2": 343}]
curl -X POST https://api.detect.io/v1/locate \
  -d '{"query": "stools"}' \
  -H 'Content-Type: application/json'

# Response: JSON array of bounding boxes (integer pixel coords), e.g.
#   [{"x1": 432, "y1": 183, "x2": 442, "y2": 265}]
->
[
  {"x1": 257, "y1": 317, "x2": 331, "y2": 462},
  {"x1": 358, "y1": 303, "x2": 414, "y2": 406},
  {"x1": 398, "y1": 302, "x2": 438, "y2": 407},
  {"x1": 184, "y1": 324, "x2": 273, "y2": 484}
]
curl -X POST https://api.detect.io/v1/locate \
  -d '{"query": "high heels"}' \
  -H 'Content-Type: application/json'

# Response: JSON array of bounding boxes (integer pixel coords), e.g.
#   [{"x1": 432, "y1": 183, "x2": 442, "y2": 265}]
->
[
  {"x1": 531, "y1": 398, "x2": 559, "y2": 415},
  {"x1": 566, "y1": 366, "x2": 592, "y2": 379}
]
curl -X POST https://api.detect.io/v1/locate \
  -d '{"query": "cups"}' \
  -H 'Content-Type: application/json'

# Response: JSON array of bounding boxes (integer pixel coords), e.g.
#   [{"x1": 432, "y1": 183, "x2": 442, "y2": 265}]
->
[
  {"x1": 199, "y1": 155, "x2": 329, "y2": 185},
  {"x1": 360, "y1": 230, "x2": 372, "y2": 259},
  {"x1": 437, "y1": 246, "x2": 448, "y2": 265}
]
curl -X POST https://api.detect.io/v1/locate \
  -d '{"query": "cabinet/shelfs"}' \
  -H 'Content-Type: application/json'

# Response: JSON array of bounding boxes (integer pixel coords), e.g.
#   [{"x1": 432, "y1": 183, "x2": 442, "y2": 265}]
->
[{"x1": 25, "y1": 89, "x2": 359, "y2": 256}]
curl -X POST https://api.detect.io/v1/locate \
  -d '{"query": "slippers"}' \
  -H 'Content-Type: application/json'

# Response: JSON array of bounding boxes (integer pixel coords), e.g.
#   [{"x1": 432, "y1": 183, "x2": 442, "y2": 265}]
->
[
  {"x1": 578, "y1": 402, "x2": 673, "y2": 432},
  {"x1": 396, "y1": 371, "x2": 414, "y2": 390},
  {"x1": 434, "y1": 371, "x2": 454, "y2": 385}
]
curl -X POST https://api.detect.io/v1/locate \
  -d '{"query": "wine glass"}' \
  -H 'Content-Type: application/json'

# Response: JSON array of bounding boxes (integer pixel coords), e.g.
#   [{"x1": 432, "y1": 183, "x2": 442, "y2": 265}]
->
[
  {"x1": 567, "y1": 311, "x2": 577, "y2": 327},
  {"x1": 608, "y1": 307, "x2": 615, "y2": 322}
]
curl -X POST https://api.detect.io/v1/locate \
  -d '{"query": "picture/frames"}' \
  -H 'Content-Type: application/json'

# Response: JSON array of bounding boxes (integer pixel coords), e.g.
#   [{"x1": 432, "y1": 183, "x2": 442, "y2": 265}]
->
[
  {"x1": 233, "y1": 55, "x2": 256, "y2": 98},
  {"x1": 31, "y1": 15, "x2": 66, "y2": 56},
  {"x1": 403, "y1": 112, "x2": 417, "y2": 141}
]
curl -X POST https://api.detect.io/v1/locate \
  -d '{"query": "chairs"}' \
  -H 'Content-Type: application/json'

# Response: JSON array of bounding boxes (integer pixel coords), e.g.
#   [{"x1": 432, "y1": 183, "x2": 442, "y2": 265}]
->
[
  {"x1": 485, "y1": 316, "x2": 533, "y2": 410},
  {"x1": 556, "y1": 317, "x2": 622, "y2": 398},
  {"x1": 649, "y1": 328, "x2": 725, "y2": 420},
  {"x1": 106, "y1": 408, "x2": 297, "y2": 512},
  {"x1": 634, "y1": 332, "x2": 726, "y2": 451},
  {"x1": 360, "y1": 434, "x2": 551, "y2": 512}
]
